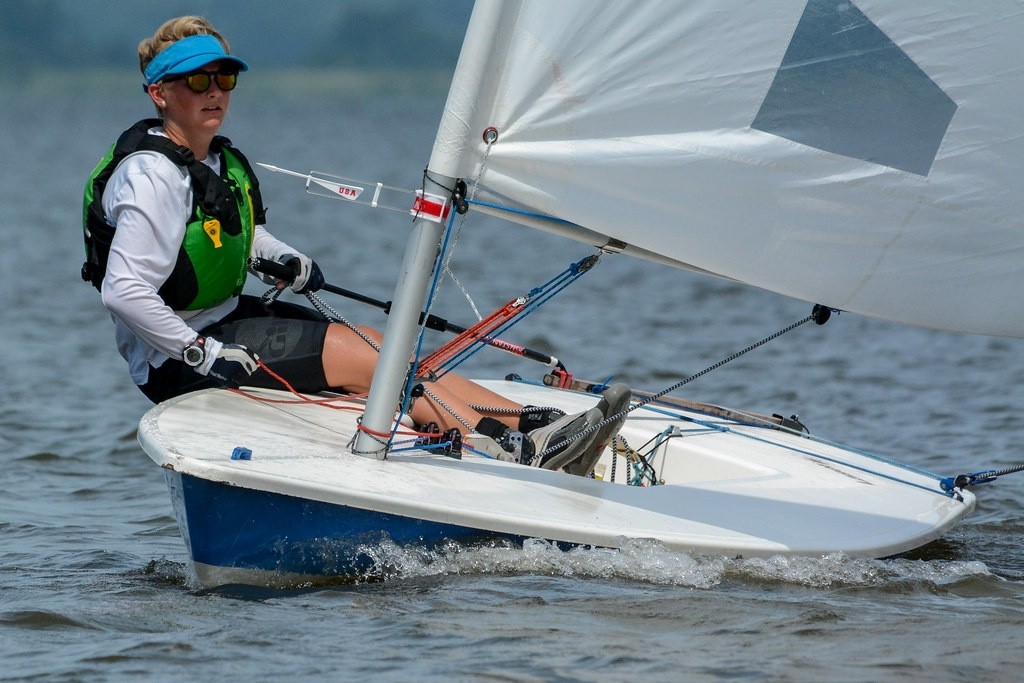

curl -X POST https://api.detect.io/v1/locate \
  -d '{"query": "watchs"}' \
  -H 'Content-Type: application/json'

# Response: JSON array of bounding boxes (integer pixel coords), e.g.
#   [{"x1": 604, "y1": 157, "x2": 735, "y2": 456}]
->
[{"x1": 182, "y1": 336, "x2": 205, "y2": 368}]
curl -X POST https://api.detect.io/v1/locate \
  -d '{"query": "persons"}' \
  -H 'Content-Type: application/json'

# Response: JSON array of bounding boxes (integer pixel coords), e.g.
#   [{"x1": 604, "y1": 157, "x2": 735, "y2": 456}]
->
[{"x1": 82, "y1": 17, "x2": 630, "y2": 476}]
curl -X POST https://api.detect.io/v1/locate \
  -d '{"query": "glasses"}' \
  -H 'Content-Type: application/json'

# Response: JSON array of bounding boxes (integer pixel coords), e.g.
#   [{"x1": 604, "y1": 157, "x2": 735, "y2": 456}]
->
[{"x1": 158, "y1": 65, "x2": 238, "y2": 94}]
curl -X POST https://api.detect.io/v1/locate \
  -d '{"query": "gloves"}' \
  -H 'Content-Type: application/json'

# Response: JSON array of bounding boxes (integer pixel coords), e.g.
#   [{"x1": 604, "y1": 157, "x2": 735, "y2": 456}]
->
[{"x1": 194, "y1": 336, "x2": 259, "y2": 391}]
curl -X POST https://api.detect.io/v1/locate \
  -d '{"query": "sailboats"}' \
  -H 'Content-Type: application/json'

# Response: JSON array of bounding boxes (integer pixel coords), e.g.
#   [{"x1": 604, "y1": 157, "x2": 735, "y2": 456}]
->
[{"x1": 134, "y1": 0, "x2": 1024, "y2": 585}]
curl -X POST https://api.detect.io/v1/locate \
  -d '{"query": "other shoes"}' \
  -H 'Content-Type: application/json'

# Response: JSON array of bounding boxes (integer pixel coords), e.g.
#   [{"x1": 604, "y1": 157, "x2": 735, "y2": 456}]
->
[
  {"x1": 505, "y1": 406, "x2": 603, "y2": 471},
  {"x1": 549, "y1": 383, "x2": 632, "y2": 478}
]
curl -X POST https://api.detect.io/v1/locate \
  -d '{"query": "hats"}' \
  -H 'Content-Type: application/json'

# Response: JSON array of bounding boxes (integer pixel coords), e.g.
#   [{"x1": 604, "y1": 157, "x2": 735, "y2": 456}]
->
[{"x1": 142, "y1": 34, "x2": 248, "y2": 93}]
